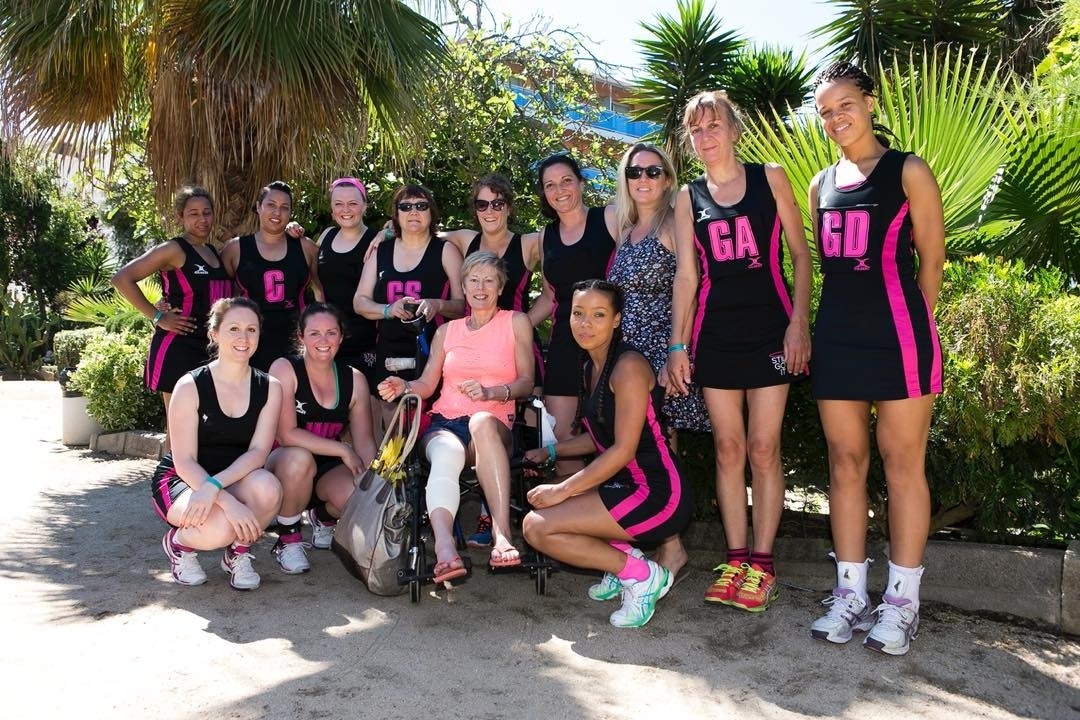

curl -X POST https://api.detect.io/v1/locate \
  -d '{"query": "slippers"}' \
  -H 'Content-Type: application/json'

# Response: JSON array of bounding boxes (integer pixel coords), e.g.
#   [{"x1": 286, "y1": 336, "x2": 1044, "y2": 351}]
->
[
  {"x1": 489, "y1": 546, "x2": 521, "y2": 567},
  {"x1": 432, "y1": 556, "x2": 467, "y2": 584}
]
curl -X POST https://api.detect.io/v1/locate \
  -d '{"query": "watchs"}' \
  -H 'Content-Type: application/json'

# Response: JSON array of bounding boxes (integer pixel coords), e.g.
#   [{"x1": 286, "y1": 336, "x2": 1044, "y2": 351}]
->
[
  {"x1": 668, "y1": 343, "x2": 689, "y2": 353},
  {"x1": 384, "y1": 303, "x2": 393, "y2": 320}
]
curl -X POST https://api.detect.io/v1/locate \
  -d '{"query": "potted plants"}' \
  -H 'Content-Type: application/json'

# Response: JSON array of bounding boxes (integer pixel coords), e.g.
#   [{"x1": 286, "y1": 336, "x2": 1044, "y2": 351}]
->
[{"x1": 53, "y1": 326, "x2": 103, "y2": 445}]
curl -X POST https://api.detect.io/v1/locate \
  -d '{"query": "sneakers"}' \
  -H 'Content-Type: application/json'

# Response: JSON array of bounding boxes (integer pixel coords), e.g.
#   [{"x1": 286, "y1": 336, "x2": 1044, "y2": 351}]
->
[
  {"x1": 809, "y1": 586, "x2": 878, "y2": 645},
  {"x1": 465, "y1": 515, "x2": 493, "y2": 548},
  {"x1": 588, "y1": 547, "x2": 649, "y2": 602},
  {"x1": 609, "y1": 559, "x2": 675, "y2": 628},
  {"x1": 220, "y1": 543, "x2": 262, "y2": 591},
  {"x1": 731, "y1": 563, "x2": 782, "y2": 613},
  {"x1": 703, "y1": 558, "x2": 750, "y2": 606},
  {"x1": 161, "y1": 526, "x2": 208, "y2": 586},
  {"x1": 861, "y1": 594, "x2": 921, "y2": 656},
  {"x1": 270, "y1": 532, "x2": 314, "y2": 574},
  {"x1": 307, "y1": 507, "x2": 337, "y2": 551}
]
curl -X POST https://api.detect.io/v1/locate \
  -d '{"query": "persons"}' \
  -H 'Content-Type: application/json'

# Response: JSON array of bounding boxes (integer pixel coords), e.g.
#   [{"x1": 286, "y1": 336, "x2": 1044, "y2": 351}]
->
[
  {"x1": 523, "y1": 279, "x2": 691, "y2": 629},
  {"x1": 111, "y1": 142, "x2": 714, "y2": 591},
  {"x1": 269, "y1": 301, "x2": 378, "y2": 575},
  {"x1": 377, "y1": 249, "x2": 535, "y2": 584},
  {"x1": 808, "y1": 60, "x2": 945, "y2": 655},
  {"x1": 666, "y1": 90, "x2": 814, "y2": 615},
  {"x1": 151, "y1": 294, "x2": 284, "y2": 591}
]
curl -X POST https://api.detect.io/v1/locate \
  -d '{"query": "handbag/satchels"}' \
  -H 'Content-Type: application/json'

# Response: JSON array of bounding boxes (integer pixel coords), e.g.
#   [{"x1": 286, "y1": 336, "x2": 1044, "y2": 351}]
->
[{"x1": 328, "y1": 392, "x2": 423, "y2": 598}]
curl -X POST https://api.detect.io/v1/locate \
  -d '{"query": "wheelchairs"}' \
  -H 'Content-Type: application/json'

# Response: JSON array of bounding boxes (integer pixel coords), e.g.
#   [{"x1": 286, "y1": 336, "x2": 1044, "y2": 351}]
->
[{"x1": 398, "y1": 392, "x2": 560, "y2": 605}]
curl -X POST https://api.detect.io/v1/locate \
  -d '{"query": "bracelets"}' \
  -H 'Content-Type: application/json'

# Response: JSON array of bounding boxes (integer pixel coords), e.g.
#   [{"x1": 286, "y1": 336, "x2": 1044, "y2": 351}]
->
[
  {"x1": 500, "y1": 384, "x2": 511, "y2": 405},
  {"x1": 153, "y1": 311, "x2": 163, "y2": 323},
  {"x1": 405, "y1": 380, "x2": 411, "y2": 395},
  {"x1": 206, "y1": 477, "x2": 223, "y2": 491},
  {"x1": 548, "y1": 444, "x2": 556, "y2": 462}
]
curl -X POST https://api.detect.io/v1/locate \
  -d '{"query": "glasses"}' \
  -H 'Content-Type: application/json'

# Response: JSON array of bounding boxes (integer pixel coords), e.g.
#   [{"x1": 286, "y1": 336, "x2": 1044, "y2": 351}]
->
[
  {"x1": 397, "y1": 201, "x2": 431, "y2": 211},
  {"x1": 473, "y1": 198, "x2": 508, "y2": 212},
  {"x1": 624, "y1": 165, "x2": 666, "y2": 180}
]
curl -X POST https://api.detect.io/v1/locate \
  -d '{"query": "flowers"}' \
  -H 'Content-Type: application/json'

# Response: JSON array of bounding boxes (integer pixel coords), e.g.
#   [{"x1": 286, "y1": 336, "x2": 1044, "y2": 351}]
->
[{"x1": 369, "y1": 439, "x2": 405, "y2": 483}]
